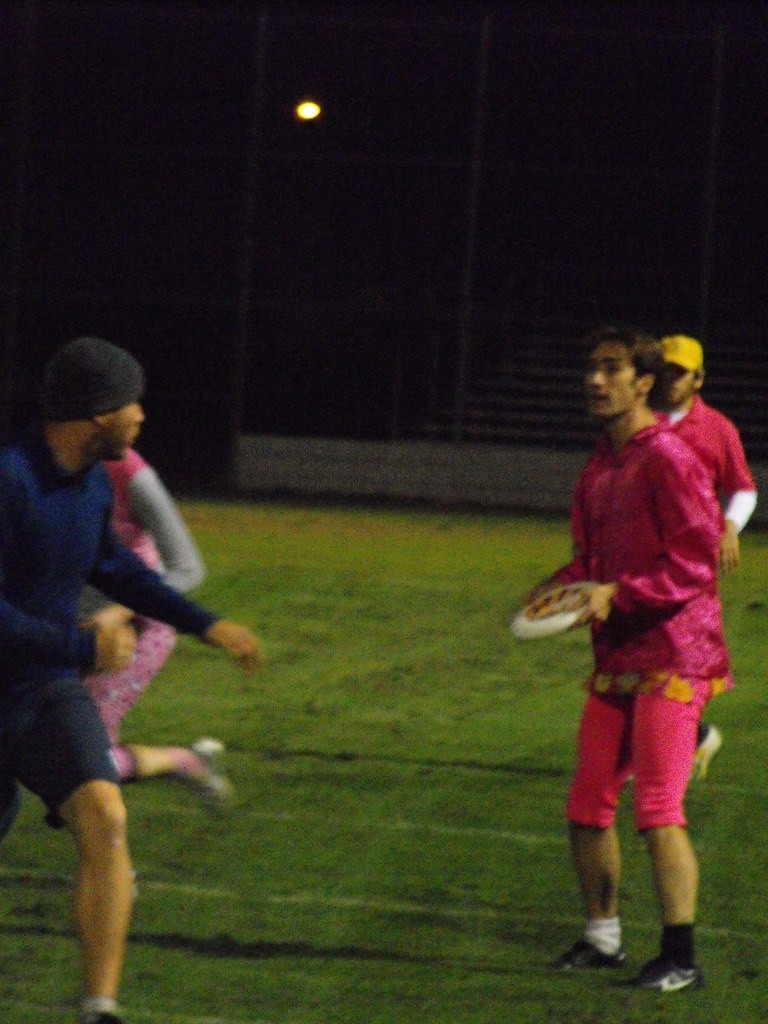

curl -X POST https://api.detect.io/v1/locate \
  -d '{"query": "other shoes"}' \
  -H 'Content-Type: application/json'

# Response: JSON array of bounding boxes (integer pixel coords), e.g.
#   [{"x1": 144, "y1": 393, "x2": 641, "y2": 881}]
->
[
  {"x1": 191, "y1": 736, "x2": 235, "y2": 819},
  {"x1": 73, "y1": 1010, "x2": 122, "y2": 1024},
  {"x1": 691, "y1": 723, "x2": 723, "y2": 781}
]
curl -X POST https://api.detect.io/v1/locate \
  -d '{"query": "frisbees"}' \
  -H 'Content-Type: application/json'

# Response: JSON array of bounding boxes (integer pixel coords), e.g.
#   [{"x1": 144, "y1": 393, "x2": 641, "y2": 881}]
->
[{"x1": 510, "y1": 580, "x2": 604, "y2": 640}]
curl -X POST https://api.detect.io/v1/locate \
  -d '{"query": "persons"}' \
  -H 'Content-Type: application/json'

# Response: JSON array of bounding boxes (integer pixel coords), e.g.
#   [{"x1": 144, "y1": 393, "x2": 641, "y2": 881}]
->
[
  {"x1": 0, "y1": 336, "x2": 257, "y2": 1024},
  {"x1": 527, "y1": 328, "x2": 727, "y2": 997},
  {"x1": 79, "y1": 448, "x2": 235, "y2": 817},
  {"x1": 649, "y1": 334, "x2": 757, "y2": 782}
]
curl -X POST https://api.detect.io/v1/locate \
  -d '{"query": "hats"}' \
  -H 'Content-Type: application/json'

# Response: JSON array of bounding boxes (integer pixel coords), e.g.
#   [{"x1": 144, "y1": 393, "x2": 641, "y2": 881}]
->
[
  {"x1": 656, "y1": 334, "x2": 704, "y2": 370},
  {"x1": 37, "y1": 335, "x2": 145, "y2": 422}
]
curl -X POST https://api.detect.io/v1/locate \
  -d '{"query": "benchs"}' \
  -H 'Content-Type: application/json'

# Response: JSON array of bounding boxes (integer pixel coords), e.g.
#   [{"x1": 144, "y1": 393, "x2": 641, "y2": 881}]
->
[{"x1": 416, "y1": 333, "x2": 768, "y2": 462}]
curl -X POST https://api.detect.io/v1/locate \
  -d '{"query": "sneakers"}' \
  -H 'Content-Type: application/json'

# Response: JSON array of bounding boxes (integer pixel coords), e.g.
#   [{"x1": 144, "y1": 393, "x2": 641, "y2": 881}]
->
[
  {"x1": 543, "y1": 936, "x2": 627, "y2": 978},
  {"x1": 612, "y1": 960, "x2": 703, "y2": 1000}
]
